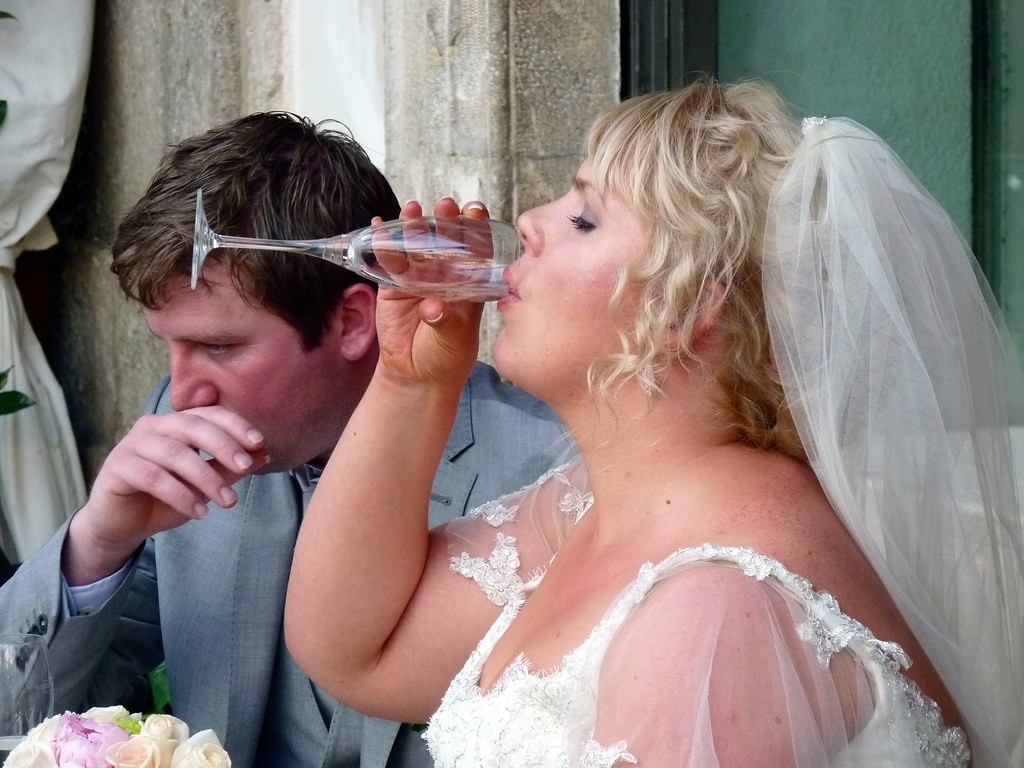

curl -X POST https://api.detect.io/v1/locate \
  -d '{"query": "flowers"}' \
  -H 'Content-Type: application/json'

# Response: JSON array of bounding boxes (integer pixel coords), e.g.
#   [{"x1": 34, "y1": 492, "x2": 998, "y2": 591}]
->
[{"x1": 2, "y1": 705, "x2": 234, "y2": 768}]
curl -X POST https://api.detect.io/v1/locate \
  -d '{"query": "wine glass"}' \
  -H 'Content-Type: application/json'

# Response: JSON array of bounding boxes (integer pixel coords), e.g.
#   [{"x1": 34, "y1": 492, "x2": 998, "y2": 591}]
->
[{"x1": 191, "y1": 189, "x2": 521, "y2": 302}]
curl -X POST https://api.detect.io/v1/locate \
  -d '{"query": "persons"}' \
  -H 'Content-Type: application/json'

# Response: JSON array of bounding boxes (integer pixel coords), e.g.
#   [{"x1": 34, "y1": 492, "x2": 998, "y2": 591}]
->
[
  {"x1": 0, "y1": 109, "x2": 584, "y2": 768},
  {"x1": 284, "y1": 80, "x2": 1024, "y2": 768}
]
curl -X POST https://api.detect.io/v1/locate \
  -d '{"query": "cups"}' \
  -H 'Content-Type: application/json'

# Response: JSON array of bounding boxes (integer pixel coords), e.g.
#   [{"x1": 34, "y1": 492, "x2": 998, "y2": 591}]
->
[{"x1": 0, "y1": 633, "x2": 54, "y2": 768}]
